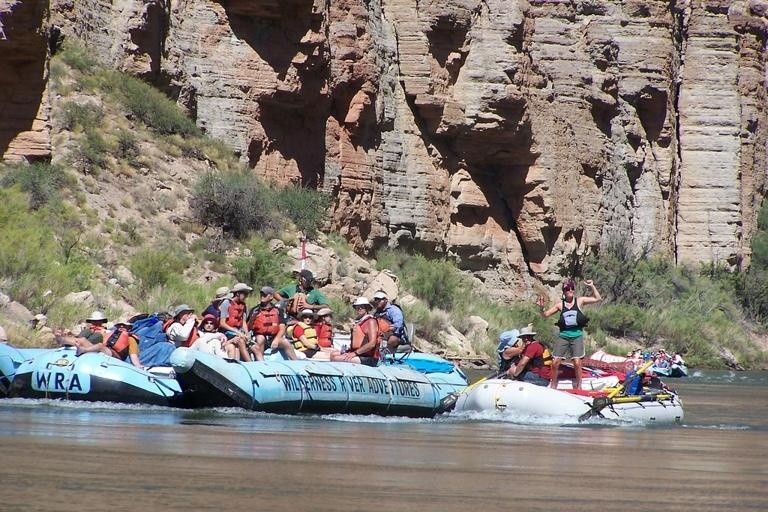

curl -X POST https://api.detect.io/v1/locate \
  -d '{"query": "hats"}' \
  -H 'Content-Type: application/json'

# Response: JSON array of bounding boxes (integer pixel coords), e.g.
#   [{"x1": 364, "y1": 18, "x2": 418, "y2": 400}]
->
[
  {"x1": 260, "y1": 286, "x2": 273, "y2": 294},
  {"x1": 114, "y1": 316, "x2": 133, "y2": 325},
  {"x1": 197, "y1": 315, "x2": 217, "y2": 328},
  {"x1": 352, "y1": 297, "x2": 373, "y2": 311},
  {"x1": 173, "y1": 304, "x2": 195, "y2": 318},
  {"x1": 562, "y1": 281, "x2": 574, "y2": 290},
  {"x1": 496, "y1": 325, "x2": 537, "y2": 353},
  {"x1": 299, "y1": 270, "x2": 315, "y2": 282},
  {"x1": 229, "y1": 283, "x2": 253, "y2": 293},
  {"x1": 374, "y1": 292, "x2": 388, "y2": 300},
  {"x1": 297, "y1": 309, "x2": 318, "y2": 321},
  {"x1": 86, "y1": 311, "x2": 108, "y2": 323},
  {"x1": 317, "y1": 308, "x2": 333, "y2": 316},
  {"x1": 128, "y1": 312, "x2": 149, "y2": 323},
  {"x1": 215, "y1": 286, "x2": 234, "y2": 300}
]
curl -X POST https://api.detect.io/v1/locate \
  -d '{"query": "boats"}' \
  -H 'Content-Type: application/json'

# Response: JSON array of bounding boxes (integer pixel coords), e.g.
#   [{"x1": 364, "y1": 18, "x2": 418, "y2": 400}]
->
[
  {"x1": 456, "y1": 366, "x2": 684, "y2": 434},
  {"x1": 651, "y1": 360, "x2": 689, "y2": 378},
  {"x1": 6, "y1": 346, "x2": 182, "y2": 405},
  {"x1": 0, "y1": 342, "x2": 56, "y2": 398},
  {"x1": 589, "y1": 348, "x2": 652, "y2": 374},
  {"x1": 170, "y1": 346, "x2": 468, "y2": 417}
]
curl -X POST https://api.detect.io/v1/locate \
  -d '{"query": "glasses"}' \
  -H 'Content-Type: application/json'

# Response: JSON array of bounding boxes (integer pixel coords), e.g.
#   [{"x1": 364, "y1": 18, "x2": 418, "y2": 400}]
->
[
  {"x1": 375, "y1": 298, "x2": 385, "y2": 301},
  {"x1": 240, "y1": 291, "x2": 249, "y2": 295},
  {"x1": 565, "y1": 286, "x2": 574, "y2": 290},
  {"x1": 355, "y1": 306, "x2": 367, "y2": 311},
  {"x1": 303, "y1": 315, "x2": 312, "y2": 319},
  {"x1": 205, "y1": 321, "x2": 213, "y2": 325},
  {"x1": 261, "y1": 293, "x2": 267, "y2": 297}
]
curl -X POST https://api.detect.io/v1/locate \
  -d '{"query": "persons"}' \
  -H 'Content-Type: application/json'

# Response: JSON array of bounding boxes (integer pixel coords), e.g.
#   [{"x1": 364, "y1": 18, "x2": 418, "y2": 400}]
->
[
  {"x1": 504, "y1": 326, "x2": 553, "y2": 386},
  {"x1": 330, "y1": 297, "x2": 381, "y2": 368},
  {"x1": 273, "y1": 270, "x2": 340, "y2": 359},
  {"x1": 57, "y1": 303, "x2": 200, "y2": 369},
  {"x1": 496, "y1": 328, "x2": 526, "y2": 380},
  {"x1": 625, "y1": 346, "x2": 685, "y2": 376},
  {"x1": 372, "y1": 291, "x2": 409, "y2": 353},
  {"x1": 197, "y1": 283, "x2": 298, "y2": 363},
  {"x1": 535, "y1": 279, "x2": 604, "y2": 390}
]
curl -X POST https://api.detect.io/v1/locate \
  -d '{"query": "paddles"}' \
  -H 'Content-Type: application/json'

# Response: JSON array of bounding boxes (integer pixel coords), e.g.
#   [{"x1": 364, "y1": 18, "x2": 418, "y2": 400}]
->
[
  {"x1": 461, "y1": 371, "x2": 499, "y2": 394},
  {"x1": 578, "y1": 360, "x2": 654, "y2": 422}
]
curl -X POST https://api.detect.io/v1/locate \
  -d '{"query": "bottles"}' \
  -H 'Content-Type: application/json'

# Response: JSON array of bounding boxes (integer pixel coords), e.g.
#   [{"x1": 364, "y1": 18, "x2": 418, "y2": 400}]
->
[
  {"x1": 340, "y1": 345, "x2": 346, "y2": 355},
  {"x1": 538, "y1": 293, "x2": 544, "y2": 313},
  {"x1": 194, "y1": 319, "x2": 198, "y2": 326}
]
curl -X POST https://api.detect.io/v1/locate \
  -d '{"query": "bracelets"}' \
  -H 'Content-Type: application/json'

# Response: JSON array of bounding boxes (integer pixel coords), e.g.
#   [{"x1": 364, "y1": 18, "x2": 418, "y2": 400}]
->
[{"x1": 354, "y1": 351, "x2": 358, "y2": 356}]
